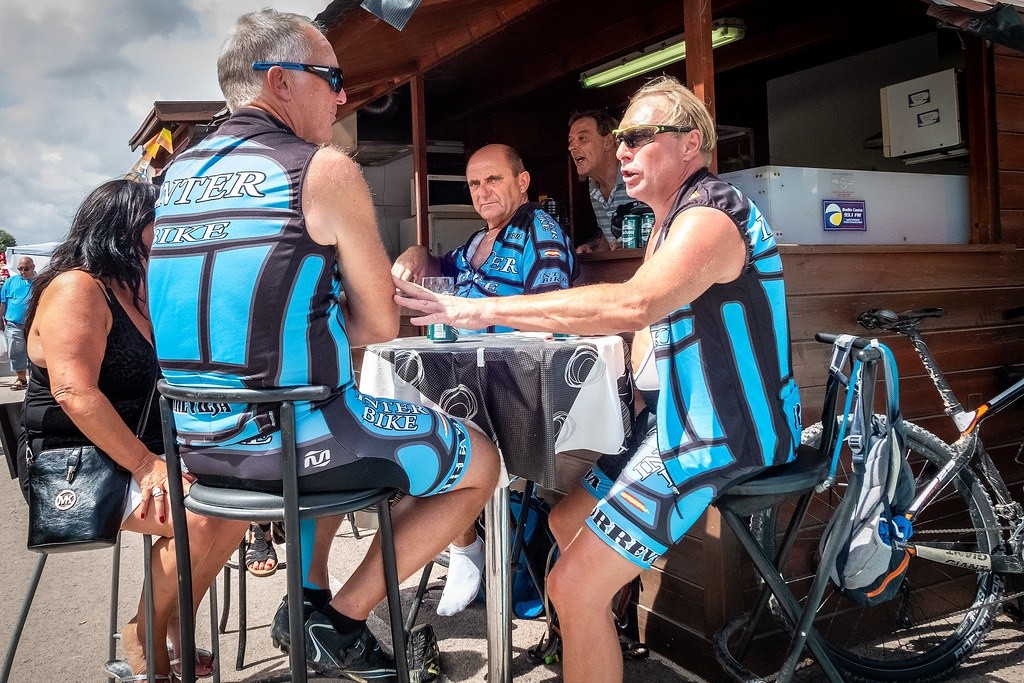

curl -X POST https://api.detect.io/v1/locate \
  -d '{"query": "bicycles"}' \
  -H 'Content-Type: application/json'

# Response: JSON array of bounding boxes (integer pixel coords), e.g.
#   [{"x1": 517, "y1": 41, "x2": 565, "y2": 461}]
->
[{"x1": 747, "y1": 306, "x2": 1023, "y2": 677}]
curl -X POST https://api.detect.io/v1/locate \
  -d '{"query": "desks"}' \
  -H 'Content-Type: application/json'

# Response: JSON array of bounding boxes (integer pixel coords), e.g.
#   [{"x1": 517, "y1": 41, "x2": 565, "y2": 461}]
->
[{"x1": 359, "y1": 333, "x2": 635, "y2": 683}]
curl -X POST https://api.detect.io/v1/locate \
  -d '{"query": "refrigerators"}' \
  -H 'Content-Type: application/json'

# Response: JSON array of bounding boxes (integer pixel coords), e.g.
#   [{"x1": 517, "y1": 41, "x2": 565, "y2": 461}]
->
[{"x1": 399, "y1": 213, "x2": 488, "y2": 261}]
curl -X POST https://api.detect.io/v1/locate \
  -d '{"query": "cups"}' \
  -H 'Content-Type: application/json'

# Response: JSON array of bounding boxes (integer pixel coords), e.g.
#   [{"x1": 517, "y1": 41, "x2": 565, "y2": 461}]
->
[{"x1": 422, "y1": 277, "x2": 454, "y2": 294}]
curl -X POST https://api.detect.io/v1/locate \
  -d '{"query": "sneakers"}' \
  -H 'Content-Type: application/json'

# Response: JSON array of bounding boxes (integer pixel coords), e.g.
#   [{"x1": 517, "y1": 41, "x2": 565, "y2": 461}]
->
[
  {"x1": 270, "y1": 596, "x2": 322, "y2": 656},
  {"x1": 303, "y1": 611, "x2": 398, "y2": 683},
  {"x1": 404, "y1": 622, "x2": 441, "y2": 683}
]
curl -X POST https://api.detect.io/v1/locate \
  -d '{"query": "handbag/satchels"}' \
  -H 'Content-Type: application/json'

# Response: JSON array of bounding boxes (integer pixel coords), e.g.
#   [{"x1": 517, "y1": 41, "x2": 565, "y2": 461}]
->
[
  {"x1": 26, "y1": 445, "x2": 135, "y2": 554},
  {"x1": 820, "y1": 339, "x2": 914, "y2": 610},
  {"x1": 474, "y1": 475, "x2": 561, "y2": 620}
]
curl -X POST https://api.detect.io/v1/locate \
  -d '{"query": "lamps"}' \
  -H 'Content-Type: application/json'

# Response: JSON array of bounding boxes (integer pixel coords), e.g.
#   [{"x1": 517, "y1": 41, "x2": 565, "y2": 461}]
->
[{"x1": 579, "y1": 14, "x2": 747, "y2": 90}]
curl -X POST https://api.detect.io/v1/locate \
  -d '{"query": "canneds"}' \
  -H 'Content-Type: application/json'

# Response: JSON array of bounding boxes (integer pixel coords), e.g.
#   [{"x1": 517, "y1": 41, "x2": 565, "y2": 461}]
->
[
  {"x1": 426, "y1": 291, "x2": 458, "y2": 343},
  {"x1": 553, "y1": 333, "x2": 579, "y2": 340},
  {"x1": 622, "y1": 215, "x2": 640, "y2": 249},
  {"x1": 639, "y1": 213, "x2": 656, "y2": 247}
]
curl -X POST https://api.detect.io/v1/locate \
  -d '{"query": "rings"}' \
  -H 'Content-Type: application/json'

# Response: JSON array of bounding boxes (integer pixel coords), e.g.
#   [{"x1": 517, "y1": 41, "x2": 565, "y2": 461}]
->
[{"x1": 152, "y1": 488, "x2": 164, "y2": 497}]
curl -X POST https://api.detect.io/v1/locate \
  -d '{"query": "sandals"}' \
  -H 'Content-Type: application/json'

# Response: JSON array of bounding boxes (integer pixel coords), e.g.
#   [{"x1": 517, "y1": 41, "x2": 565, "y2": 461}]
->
[
  {"x1": 10, "y1": 378, "x2": 28, "y2": 391},
  {"x1": 272, "y1": 520, "x2": 287, "y2": 546},
  {"x1": 245, "y1": 519, "x2": 278, "y2": 576}
]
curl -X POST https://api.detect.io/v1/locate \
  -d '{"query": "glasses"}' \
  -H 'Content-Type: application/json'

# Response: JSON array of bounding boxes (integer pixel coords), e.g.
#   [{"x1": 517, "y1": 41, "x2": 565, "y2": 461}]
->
[
  {"x1": 252, "y1": 61, "x2": 344, "y2": 93},
  {"x1": 612, "y1": 125, "x2": 696, "y2": 149},
  {"x1": 17, "y1": 264, "x2": 33, "y2": 271}
]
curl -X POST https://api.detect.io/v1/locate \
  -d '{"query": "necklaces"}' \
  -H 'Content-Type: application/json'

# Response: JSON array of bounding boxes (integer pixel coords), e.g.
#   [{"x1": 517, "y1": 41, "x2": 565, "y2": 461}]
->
[{"x1": 484, "y1": 236, "x2": 496, "y2": 241}]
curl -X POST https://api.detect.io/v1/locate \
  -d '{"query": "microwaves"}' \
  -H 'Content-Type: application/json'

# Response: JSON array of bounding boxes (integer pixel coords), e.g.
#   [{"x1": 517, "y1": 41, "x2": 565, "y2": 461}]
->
[{"x1": 409, "y1": 174, "x2": 477, "y2": 213}]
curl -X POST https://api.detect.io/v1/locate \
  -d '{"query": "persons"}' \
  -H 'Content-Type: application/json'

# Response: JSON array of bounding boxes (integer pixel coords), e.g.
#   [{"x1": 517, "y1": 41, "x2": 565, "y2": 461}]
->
[
  {"x1": 14, "y1": 180, "x2": 285, "y2": 683},
  {"x1": 392, "y1": 144, "x2": 577, "y2": 616},
  {"x1": 0, "y1": 257, "x2": 40, "y2": 391},
  {"x1": 567, "y1": 110, "x2": 653, "y2": 254},
  {"x1": 391, "y1": 81, "x2": 801, "y2": 683},
  {"x1": 145, "y1": 14, "x2": 501, "y2": 683}
]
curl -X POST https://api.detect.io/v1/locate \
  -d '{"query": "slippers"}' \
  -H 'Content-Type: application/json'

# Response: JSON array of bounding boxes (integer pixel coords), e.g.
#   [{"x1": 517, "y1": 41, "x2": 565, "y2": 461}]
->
[
  {"x1": 165, "y1": 641, "x2": 217, "y2": 678},
  {"x1": 103, "y1": 660, "x2": 174, "y2": 683}
]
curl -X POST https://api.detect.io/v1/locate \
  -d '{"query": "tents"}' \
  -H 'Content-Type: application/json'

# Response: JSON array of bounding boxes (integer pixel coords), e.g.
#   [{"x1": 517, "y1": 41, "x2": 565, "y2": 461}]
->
[{"x1": 5, "y1": 241, "x2": 65, "y2": 278}]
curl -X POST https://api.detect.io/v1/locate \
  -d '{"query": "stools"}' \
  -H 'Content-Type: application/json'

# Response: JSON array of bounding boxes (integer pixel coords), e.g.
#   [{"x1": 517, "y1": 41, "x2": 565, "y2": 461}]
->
[
  {"x1": 0, "y1": 402, "x2": 222, "y2": 683},
  {"x1": 709, "y1": 332, "x2": 883, "y2": 683},
  {"x1": 156, "y1": 378, "x2": 411, "y2": 683}
]
